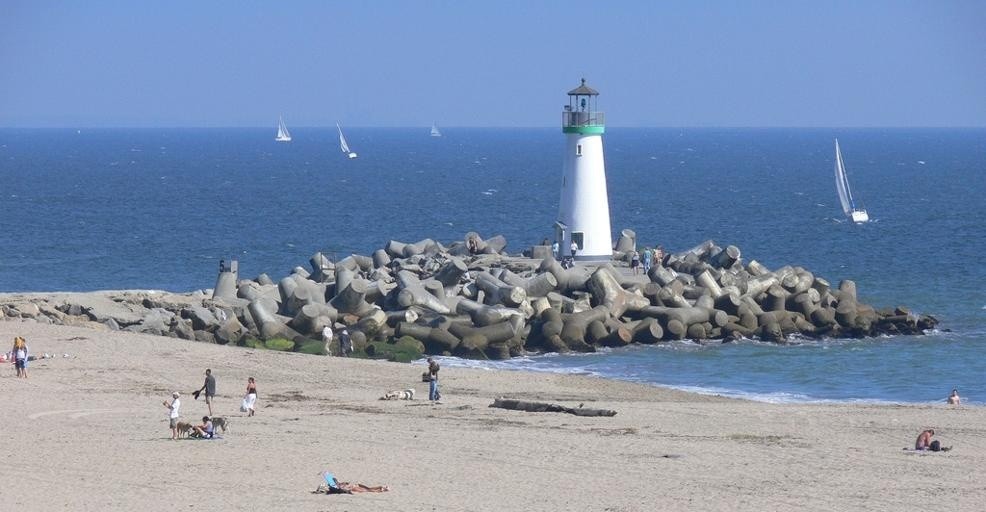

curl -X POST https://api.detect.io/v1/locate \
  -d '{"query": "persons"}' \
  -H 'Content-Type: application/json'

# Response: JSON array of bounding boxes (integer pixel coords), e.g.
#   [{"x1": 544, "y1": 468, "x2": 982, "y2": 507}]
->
[
  {"x1": 570, "y1": 240, "x2": 577, "y2": 261},
  {"x1": 189, "y1": 416, "x2": 213, "y2": 440},
  {"x1": 653, "y1": 245, "x2": 658, "y2": 263},
  {"x1": 337, "y1": 327, "x2": 353, "y2": 357},
  {"x1": 327, "y1": 477, "x2": 390, "y2": 494},
  {"x1": 425, "y1": 357, "x2": 443, "y2": 400},
  {"x1": 630, "y1": 251, "x2": 639, "y2": 276},
  {"x1": 914, "y1": 428, "x2": 952, "y2": 452},
  {"x1": 551, "y1": 239, "x2": 559, "y2": 259},
  {"x1": 947, "y1": 388, "x2": 961, "y2": 405},
  {"x1": 321, "y1": 325, "x2": 334, "y2": 357},
  {"x1": 542, "y1": 237, "x2": 550, "y2": 246},
  {"x1": 242, "y1": 376, "x2": 257, "y2": 416},
  {"x1": 11, "y1": 335, "x2": 28, "y2": 379},
  {"x1": 658, "y1": 243, "x2": 664, "y2": 259},
  {"x1": 427, "y1": 361, "x2": 439, "y2": 401},
  {"x1": 196, "y1": 367, "x2": 215, "y2": 416},
  {"x1": 161, "y1": 391, "x2": 181, "y2": 441},
  {"x1": 641, "y1": 246, "x2": 652, "y2": 276}
]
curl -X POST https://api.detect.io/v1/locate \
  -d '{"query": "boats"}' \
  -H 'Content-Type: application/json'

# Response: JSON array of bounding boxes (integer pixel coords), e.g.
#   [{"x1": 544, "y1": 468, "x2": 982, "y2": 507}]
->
[
  {"x1": 275, "y1": 115, "x2": 291, "y2": 141},
  {"x1": 835, "y1": 138, "x2": 869, "y2": 225},
  {"x1": 336, "y1": 121, "x2": 357, "y2": 159},
  {"x1": 430, "y1": 120, "x2": 441, "y2": 136}
]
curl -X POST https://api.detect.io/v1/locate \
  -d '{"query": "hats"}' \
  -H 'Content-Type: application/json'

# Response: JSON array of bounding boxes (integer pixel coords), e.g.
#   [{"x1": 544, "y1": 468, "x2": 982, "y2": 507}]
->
[
  {"x1": 342, "y1": 330, "x2": 348, "y2": 335},
  {"x1": 173, "y1": 392, "x2": 180, "y2": 397}
]
